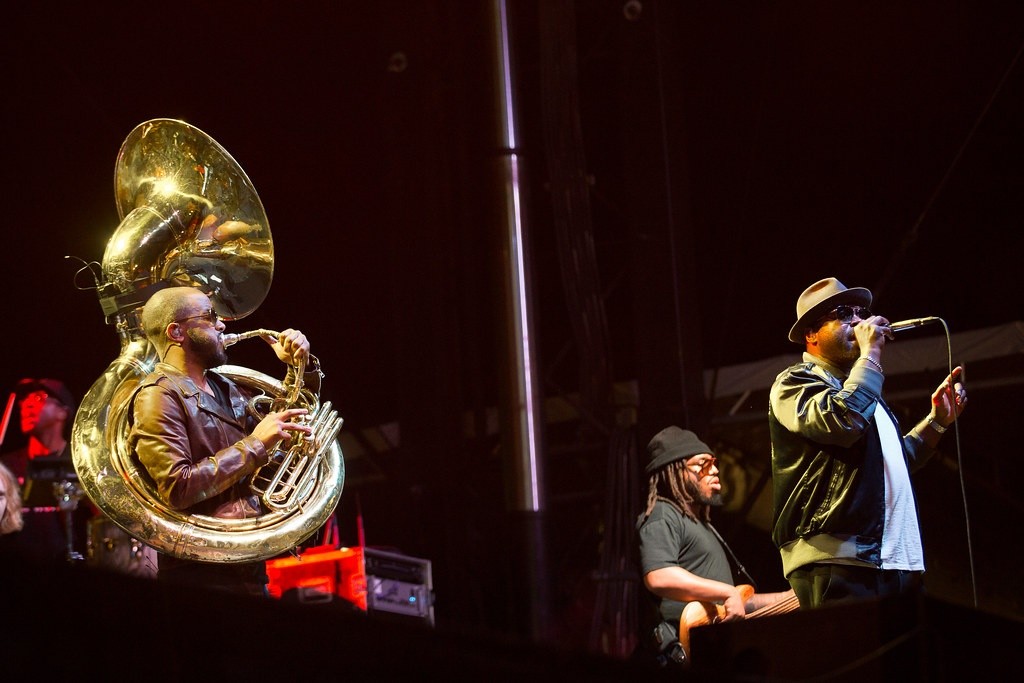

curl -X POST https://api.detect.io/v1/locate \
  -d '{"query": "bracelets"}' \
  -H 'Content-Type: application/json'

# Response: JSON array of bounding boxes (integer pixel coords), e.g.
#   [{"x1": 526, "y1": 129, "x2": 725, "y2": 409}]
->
[
  {"x1": 926, "y1": 413, "x2": 947, "y2": 434},
  {"x1": 854, "y1": 356, "x2": 883, "y2": 374}
]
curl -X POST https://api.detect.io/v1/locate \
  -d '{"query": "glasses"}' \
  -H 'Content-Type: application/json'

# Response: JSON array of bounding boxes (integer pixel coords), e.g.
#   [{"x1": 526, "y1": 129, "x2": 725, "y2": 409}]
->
[
  {"x1": 814, "y1": 300, "x2": 873, "y2": 334},
  {"x1": 681, "y1": 453, "x2": 719, "y2": 476},
  {"x1": 166, "y1": 306, "x2": 221, "y2": 330}
]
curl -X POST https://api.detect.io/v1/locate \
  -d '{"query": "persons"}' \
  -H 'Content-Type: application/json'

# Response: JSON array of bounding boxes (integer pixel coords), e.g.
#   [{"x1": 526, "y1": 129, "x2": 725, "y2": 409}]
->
[
  {"x1": 770, "y1": 277, "x2": 969, "y2": 613},
  {"x1": 633, "y1": 426, "x2": 797, "y2": 683},
  {"x1": 124, "y1": 286, "x2": 327, "y2": 600},
  {"x1": 0, "y1": 376, "x2": 85, "y2": 567}
]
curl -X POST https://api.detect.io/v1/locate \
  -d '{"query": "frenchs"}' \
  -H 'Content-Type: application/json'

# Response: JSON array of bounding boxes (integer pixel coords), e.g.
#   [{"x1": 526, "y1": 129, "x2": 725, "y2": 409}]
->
[{"x1": 69, "y1": 118, "x2": 345, "y2": 563}]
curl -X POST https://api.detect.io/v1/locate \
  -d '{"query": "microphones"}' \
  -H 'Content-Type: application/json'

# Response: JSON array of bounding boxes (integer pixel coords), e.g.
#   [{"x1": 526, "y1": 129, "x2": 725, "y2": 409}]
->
[{"x1": 881, "y1": 316, "x2": 940, "y2": 336}]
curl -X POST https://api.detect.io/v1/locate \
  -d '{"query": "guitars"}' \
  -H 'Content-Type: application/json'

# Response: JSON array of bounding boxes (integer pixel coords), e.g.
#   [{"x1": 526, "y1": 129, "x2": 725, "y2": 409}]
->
[{"x1": 652, "y1": 588, "x2": 800, "y2": 669}]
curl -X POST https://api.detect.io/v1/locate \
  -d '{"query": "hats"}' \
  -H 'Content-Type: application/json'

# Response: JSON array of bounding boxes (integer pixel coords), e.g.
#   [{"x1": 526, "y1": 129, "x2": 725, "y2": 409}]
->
[
  {"x1": 787, "y1": 276, "x2": 873, "y2": 349},
  {"x1": 14, "y1": 376, "x2": 79, "y2": 415},
  {"x1": 647, "y1": 427, "x2": 715, "y2": 475}
]
